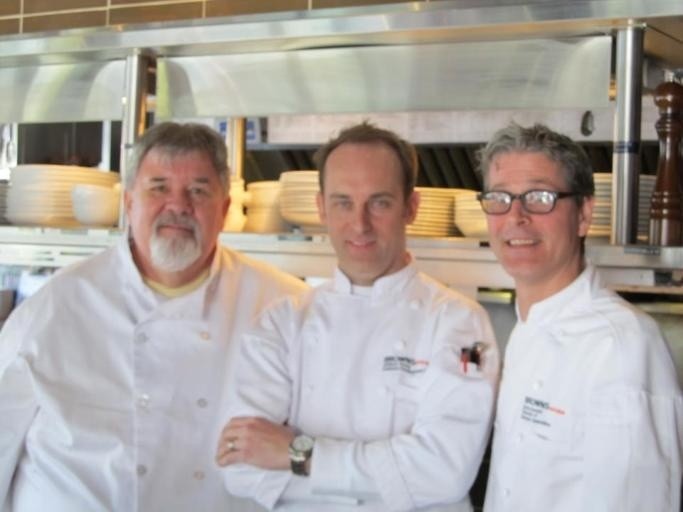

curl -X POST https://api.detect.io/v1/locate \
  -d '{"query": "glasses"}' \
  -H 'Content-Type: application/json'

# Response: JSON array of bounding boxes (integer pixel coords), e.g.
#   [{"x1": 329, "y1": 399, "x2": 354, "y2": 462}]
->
[{"x1": 475, "y1": 189, "x2": 579, "y2": 215}]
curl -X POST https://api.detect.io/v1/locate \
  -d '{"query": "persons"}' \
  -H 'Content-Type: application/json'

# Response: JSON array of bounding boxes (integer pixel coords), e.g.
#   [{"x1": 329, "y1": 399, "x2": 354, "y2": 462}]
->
[
  {"x1": 477, "y1": 120, "x2": 682, "y2": 512},
  {"x1": 0, "y1": 124, "x2": 311, "y2": 512},
  {"x1": 215, "y1": 121, "x2": 500, "y2": 512}
]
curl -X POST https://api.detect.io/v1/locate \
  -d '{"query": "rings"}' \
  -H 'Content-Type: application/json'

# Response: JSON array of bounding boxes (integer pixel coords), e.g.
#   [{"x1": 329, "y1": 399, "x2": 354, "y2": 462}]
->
[{"x1": 228, "y1": 438, "x2": 235, "y2": 453}]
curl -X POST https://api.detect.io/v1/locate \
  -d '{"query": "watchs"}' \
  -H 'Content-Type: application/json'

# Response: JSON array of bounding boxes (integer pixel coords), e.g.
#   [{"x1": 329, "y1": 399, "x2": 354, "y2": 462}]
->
[{"x1": 289, "y1": 433, "x2": 313, "y2": 477}]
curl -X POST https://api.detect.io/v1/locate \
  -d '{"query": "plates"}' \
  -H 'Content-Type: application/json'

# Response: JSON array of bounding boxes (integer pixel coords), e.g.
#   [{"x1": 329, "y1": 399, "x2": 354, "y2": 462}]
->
[{"x1": 1, "y1": 165, "x2": 658, "y2": 241}]
